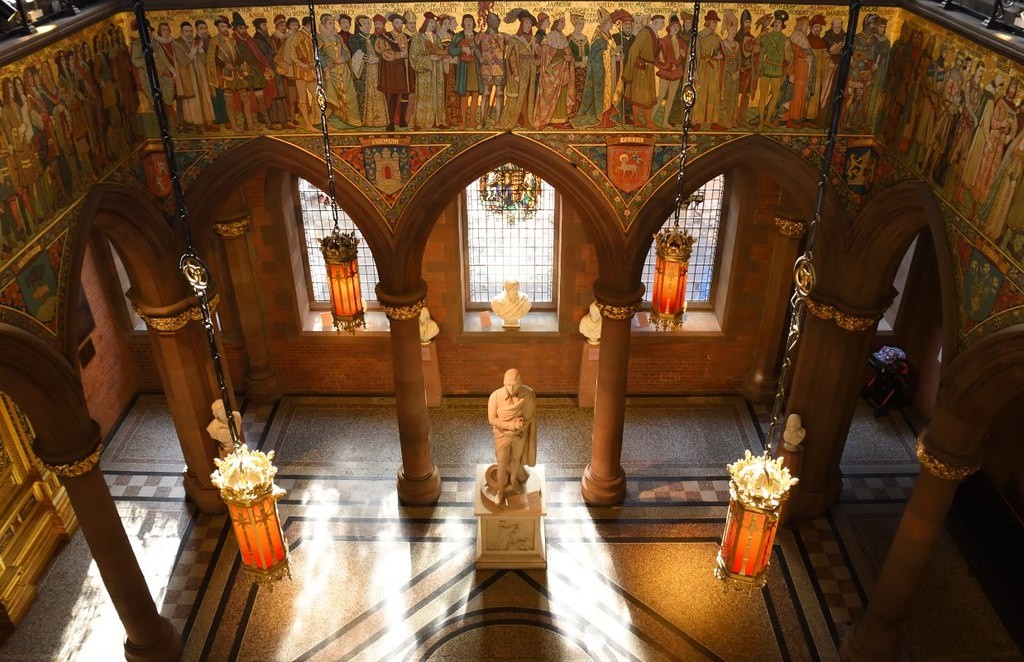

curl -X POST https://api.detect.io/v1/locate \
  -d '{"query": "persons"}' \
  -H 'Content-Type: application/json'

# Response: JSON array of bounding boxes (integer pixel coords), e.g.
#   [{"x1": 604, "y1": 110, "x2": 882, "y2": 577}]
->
[
  {"x1": 488, "y1": 368, "x2": 537, "y2": 506},
  {"x1": 206, "y1": 399, "x2": 243, "y2": 451},
  {"x1": 491, "y1": 280, "x2": 532, "y2": 323},
  {"x1": 783, "y1": 414, "x2": 806, "y2": 448},
  {"x1": 418, "y1": 307, "x2": 440, "y2": 342},
  {"x1": 578, "y1": 303, "x2": 603, "y2": 341}
]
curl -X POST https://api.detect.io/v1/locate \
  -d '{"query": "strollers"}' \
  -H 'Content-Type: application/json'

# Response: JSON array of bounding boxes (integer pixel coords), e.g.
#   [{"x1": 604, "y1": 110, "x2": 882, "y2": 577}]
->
[{"x1": 860, "y1": 346, "x2": 917, "y2": 417}]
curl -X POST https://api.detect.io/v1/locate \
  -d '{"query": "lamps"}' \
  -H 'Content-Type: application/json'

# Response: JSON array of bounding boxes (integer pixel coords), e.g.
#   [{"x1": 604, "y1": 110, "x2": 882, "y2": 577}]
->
[
  {"x1": 132, "y1": 0, "x2": 294, "y2": 595},
  {"x1": 646, "y1": 1, "x2": 700, "y2": 331},
  {"x1": 712, "y1": 1, "x2": 861, "y2": 606},
  {"x1": 307, "y1": 0, "x2": 368, "y2": 335}
]
